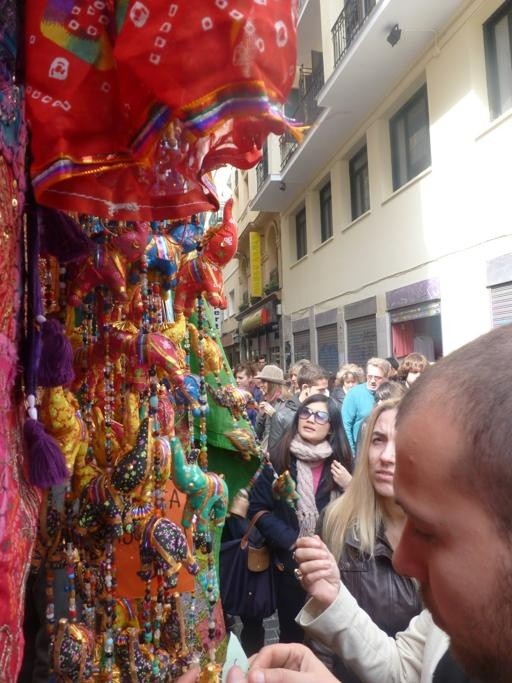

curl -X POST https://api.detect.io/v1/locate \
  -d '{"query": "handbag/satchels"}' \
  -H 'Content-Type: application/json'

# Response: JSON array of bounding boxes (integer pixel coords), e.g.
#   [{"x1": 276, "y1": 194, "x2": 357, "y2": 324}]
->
[{"x1": 219, "y1": 511, "x2": 280, "y2": 626}]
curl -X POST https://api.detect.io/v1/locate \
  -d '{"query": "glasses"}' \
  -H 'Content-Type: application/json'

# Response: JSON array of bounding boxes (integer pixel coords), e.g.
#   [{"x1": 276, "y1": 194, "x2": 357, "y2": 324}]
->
[{"x1": 297, "y1": 405, "x2": 333, "y2": 425}]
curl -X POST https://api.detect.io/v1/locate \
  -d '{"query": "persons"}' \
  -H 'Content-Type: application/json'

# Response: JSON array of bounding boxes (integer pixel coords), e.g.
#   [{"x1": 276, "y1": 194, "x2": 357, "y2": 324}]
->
[
  {"x1": 221, "y1": 352, "x2": 429, "y2": 682},
  {"x1": 225, "y1": 325, "x2": 512, "y2": 683}
]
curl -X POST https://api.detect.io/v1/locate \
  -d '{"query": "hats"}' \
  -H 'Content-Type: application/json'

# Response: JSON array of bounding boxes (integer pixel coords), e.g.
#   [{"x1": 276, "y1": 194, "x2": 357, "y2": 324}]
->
[{"x1": 252, "y1": 364, "x2": 287, "y2": 385}]
